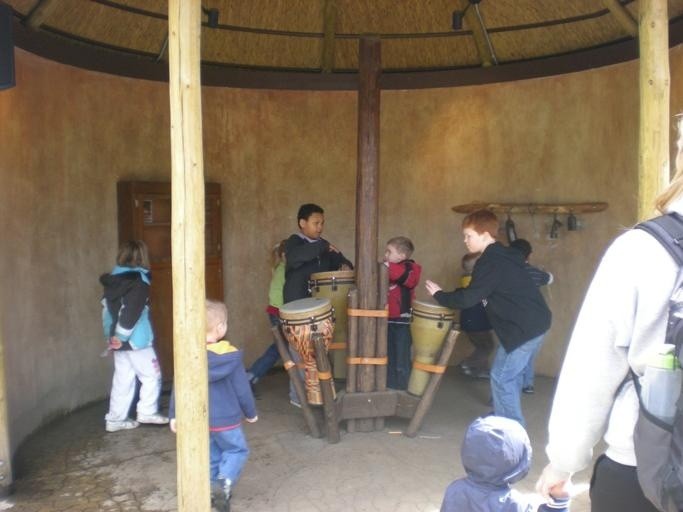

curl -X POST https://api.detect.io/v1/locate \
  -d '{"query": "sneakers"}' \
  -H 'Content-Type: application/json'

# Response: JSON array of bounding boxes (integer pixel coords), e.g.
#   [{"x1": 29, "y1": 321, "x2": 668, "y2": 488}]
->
[
  {"x1": 210, "y1": 473, "x2": 233, "y2": 512},
  {"x1": 106, "y1": 418, "x2": 140, "y2": 432},
  {"x1": 135, "y1": 413, "x2": 169, "y2": 425},
  {"x1": 249, "y1": 381, "x2": 263, "y2": 398},
  {"x1": 290, "y1": 399, "x2": 302, "y2": 409}
]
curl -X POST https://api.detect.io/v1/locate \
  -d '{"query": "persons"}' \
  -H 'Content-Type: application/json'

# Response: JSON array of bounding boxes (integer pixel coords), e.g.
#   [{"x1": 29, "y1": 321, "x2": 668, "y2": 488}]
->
[
  {"x1": 532, "y1": 113, "x2": 683, "y2": 512},
  {"x1": 438, "y1": 413, "x2": 571, "y2": 512},
  {"x1": 279, "y1": 203, "x2": 355, "y2": 408},
  {"x1": 96, "y1": 237, "x2": 170, "y2": 433},
  {"x1": 162, "y1": 296, "x2": 258, "y2": 512},
  {"x1": 382, "y1": 236, "x2": 422, "y2": 390},
  {"x1": 245, "y1": 242, "x2": 289, "y2": 399},
  {"x1": 425, "y1": 208, "x2": 555, "y2": 431}
]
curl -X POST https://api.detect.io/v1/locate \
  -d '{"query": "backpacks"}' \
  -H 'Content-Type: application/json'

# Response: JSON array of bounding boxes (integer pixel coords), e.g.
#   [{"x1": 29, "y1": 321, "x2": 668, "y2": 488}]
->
[{"x1": 629, "y1": 211, "x2": 681, "y2": 512}]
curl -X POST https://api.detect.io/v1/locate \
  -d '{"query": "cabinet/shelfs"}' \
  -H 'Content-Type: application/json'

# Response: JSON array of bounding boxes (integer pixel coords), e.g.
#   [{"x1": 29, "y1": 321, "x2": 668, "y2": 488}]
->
[{"x1": 115, "y1": 178, "x2": 229, "y2": 396}]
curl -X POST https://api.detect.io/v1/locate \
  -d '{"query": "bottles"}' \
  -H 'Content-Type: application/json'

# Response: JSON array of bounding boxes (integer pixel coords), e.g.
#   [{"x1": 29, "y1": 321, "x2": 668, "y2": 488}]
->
[{"x1": 641, "y1": 344, "x2": 683, "y2": 425}]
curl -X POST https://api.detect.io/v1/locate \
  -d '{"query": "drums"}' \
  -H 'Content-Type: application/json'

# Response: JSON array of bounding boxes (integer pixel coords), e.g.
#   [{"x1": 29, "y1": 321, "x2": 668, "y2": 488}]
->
[
  {"x1": 279, "y1": 297, "x2": 337, "y2": 405},
  {"x1": 309, "y1": 271, "x2": 358, "y2": 379},
  {"x1": 408, "y1": 296, "x2": 455, "y2": 396}
]
